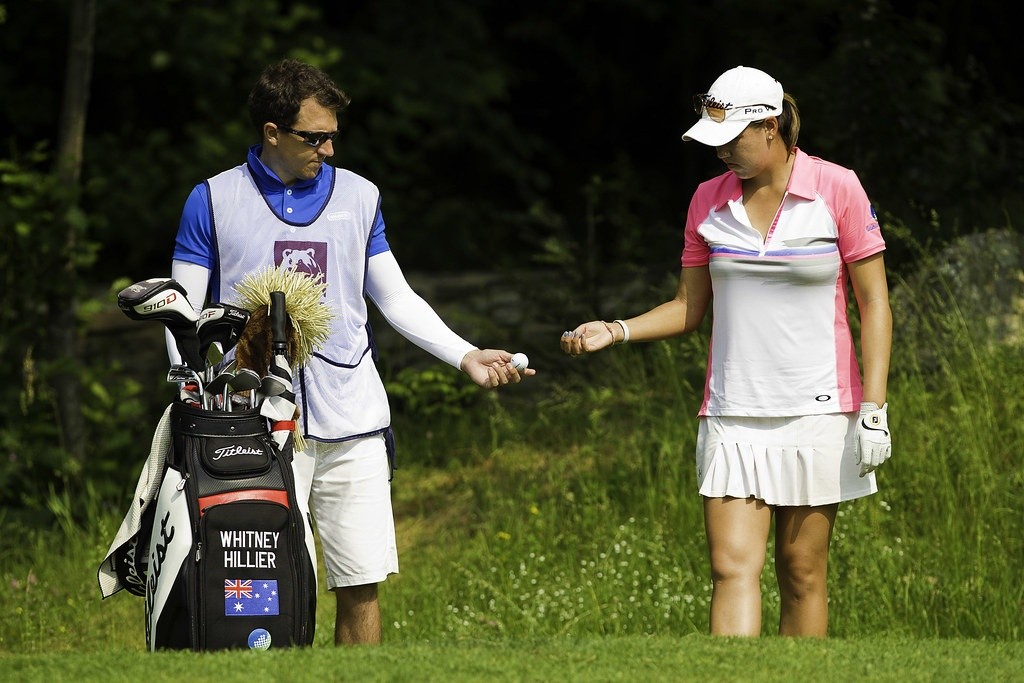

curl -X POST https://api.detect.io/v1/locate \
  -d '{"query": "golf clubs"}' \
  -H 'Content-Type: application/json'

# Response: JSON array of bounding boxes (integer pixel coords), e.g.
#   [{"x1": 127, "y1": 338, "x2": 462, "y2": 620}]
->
[{"x1": 117, "y1": 278, "x2": 286, "y2": 412}]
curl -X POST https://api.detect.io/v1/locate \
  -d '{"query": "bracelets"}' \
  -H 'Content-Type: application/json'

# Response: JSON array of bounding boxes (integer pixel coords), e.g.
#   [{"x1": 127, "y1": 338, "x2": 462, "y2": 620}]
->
[
  {"x1": 613, "y1": 319, "x2": 629, "y2": 345},
  {"x1": 602, "y1": 320, "x2": 615, "y2": 347}
]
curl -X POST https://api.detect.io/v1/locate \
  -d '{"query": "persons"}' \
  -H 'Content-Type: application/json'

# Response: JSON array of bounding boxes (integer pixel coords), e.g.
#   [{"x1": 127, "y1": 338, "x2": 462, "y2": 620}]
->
[
  {"x1": 164, "y1": 54, "x2": 535, "y2": 646},
  {"x1": 562, "y1": 64, "x2": 893, "y2": 640}
]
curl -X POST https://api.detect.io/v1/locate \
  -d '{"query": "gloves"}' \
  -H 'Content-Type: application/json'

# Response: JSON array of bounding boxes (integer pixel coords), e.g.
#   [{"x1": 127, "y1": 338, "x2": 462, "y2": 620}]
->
[{"x1": 853, "y1": 401, "x2": 891, "y2": 477}]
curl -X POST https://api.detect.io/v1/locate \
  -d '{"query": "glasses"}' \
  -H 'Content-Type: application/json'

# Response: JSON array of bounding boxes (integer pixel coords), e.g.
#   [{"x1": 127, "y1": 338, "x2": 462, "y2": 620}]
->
[
  {"x1": 274, "y1": 124, "x2": 339, "y2": 146},
  {"x1": 692, "y1": 93, "x2": 777, "y2": 124}
]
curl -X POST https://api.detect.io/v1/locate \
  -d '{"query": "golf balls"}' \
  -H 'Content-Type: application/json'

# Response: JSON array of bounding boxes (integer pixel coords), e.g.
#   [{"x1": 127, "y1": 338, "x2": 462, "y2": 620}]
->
[{"x1": 511, "y1": 353, "x2": 529, "y2": 371}]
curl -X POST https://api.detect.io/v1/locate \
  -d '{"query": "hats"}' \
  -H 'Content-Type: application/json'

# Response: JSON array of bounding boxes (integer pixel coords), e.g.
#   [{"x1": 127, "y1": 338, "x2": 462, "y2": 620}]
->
[{"x1": 681, "y1": 66, "x2": 784, "y2": 147}]
